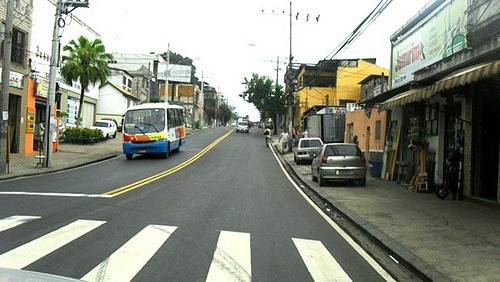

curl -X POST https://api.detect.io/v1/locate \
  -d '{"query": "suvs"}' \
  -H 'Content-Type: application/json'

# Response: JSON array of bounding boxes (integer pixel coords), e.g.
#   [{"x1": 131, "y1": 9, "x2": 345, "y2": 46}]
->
[{"x1": 236, "y1": 121, "x2": 249, "y2": 133}]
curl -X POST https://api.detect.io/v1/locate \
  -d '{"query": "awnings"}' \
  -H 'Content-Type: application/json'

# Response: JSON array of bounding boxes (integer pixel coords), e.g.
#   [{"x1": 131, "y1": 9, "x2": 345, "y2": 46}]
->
[
  {"x1": 377, "y1": 82, "x2": 434, "y2": 114},
  {"x1": 433, "y1": 60, "x2": 500, "y2": 94}
]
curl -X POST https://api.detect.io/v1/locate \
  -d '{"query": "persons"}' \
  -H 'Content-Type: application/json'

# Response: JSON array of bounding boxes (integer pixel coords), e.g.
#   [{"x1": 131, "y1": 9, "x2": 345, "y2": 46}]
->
[
  {"x1": 278, "y1": 129, "x2": 289, "y2": 153},
  {"x1": 264, "y1": 125, "x2": 271, "y2": 147},
  {"x1": 302, "y1": 129, "x2": 309, "y2": 138},
  {"x1": 349, "y1": 134, "x2": 358, "y2": 145}
]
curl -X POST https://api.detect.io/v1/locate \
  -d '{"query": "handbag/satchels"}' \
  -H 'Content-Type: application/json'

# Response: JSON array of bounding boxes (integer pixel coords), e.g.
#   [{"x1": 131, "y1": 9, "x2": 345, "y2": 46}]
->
[{"x1": 264, "y1": 130, "x2": 269, "y2": 135}]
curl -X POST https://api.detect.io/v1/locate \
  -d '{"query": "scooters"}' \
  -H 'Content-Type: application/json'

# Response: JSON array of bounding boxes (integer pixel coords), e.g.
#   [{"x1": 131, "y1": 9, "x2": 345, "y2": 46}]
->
[{"x1": 435, "y1": 165, "x2": 462, "y2": 199}]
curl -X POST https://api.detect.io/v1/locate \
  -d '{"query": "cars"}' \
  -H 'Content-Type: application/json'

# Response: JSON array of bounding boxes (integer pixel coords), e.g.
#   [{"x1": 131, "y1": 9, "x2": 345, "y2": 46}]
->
[
  {"x1": 291, "y1": 137, "x2": 324, "y2": 164},
  {"x1": 34, "y1": 120, "x2": 66, "y2": 150},
  {"x1": 91, "y1": 118, "x2": 121, "y2": 140},
  {"x1": 311, "y1": 143, "x2": 367, "y2": 187}
]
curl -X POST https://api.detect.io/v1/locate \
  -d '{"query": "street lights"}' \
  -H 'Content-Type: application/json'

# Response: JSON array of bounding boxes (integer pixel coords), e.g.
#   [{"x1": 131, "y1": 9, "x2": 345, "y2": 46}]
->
[{"x1": 164, "y1": 57, "x2": 201, "y2": 102}]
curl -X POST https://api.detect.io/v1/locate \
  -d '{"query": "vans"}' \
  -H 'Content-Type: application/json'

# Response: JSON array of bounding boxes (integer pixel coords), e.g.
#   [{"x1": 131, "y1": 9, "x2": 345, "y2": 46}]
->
[{"x1": 122, "y1": 102, "x2": 186, "y2": 160}]
